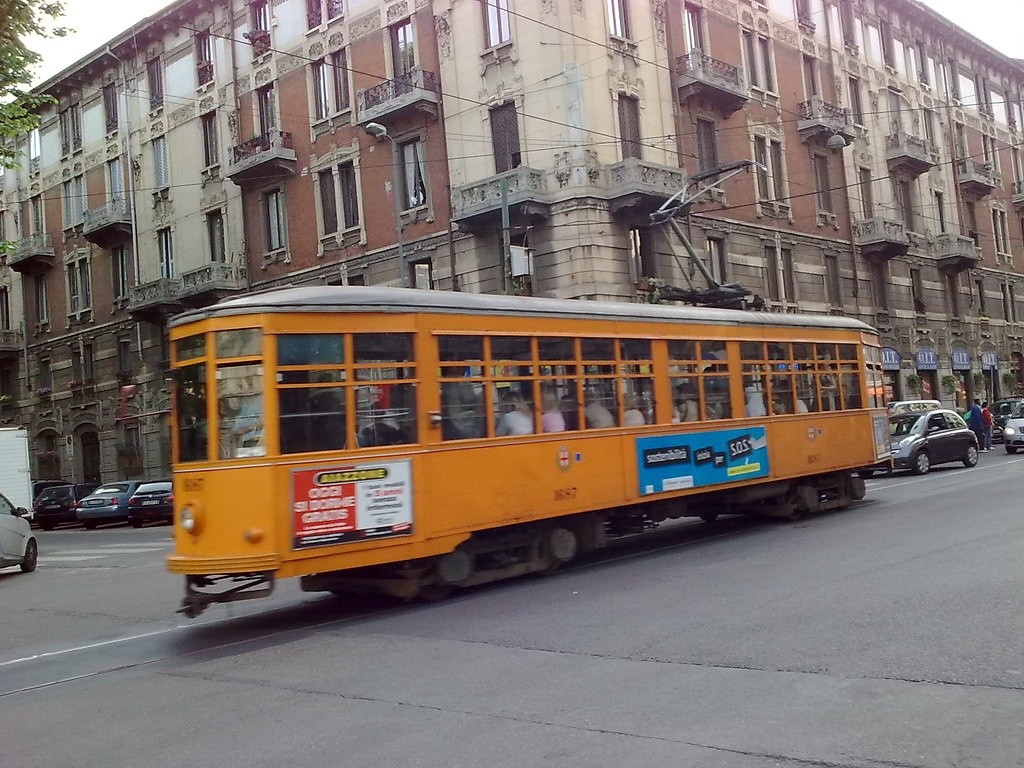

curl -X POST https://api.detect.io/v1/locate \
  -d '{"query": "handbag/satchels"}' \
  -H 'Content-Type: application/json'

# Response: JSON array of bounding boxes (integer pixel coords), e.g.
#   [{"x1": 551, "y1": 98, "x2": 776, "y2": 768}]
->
[{"x1": 963, "y1": 410, "x2": 972, "y2": 421}]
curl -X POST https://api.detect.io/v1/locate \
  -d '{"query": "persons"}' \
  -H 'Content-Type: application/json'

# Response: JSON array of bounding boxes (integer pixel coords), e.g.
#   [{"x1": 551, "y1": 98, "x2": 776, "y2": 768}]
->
[
  {"x1": 971, "y1": 399, "x2": 989, "y2": 453},
  {"x1": 275, "y1": 356, "x2": 850, "y2": 454},
  {"x1": 982, "y1": 402, "x2": 996, "y2": 450}
]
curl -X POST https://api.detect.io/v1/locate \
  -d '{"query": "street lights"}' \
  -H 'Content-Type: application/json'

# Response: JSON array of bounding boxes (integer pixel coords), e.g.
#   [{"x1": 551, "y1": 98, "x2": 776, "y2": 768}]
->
[{"x1": 367, "y1": 121, "x2": 406, "y2": 287}]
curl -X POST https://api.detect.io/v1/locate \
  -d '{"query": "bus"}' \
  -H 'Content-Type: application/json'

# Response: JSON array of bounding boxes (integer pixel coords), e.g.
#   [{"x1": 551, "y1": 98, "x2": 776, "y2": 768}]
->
[{"x1": 165, "y1": 281, "x2": 899, "y2": 620}]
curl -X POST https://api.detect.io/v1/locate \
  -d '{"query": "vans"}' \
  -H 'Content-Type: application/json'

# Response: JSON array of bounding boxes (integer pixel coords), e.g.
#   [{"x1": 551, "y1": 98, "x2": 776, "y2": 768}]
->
[{"x1": 885, "y1": 399, "x2": 942, "y2": 434}]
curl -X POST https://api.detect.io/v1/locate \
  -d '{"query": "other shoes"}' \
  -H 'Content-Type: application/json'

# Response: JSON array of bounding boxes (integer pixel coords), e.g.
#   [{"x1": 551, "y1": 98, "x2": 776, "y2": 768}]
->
[
  {"x1": 983, "y1": 447, "x2": 987, "y2": 451},
  {"x1": 988, "y1": 447, "x2": 996, "y2": 450},
  {"x1": 977, "y1": 449, "x2": 989, "y2": 453}
]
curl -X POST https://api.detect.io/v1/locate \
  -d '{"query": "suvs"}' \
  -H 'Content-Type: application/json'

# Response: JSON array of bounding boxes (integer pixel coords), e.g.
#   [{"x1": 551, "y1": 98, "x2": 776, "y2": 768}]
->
[
  {"x1": 31, "y1": 483, "x2": 103, "y2": 531},
  {"x1": 967, "y1": 399, "x2": 1021, "y2": 445},
  {"x1": 31, "y1": 479, "x2": 74, "y2": 505}
]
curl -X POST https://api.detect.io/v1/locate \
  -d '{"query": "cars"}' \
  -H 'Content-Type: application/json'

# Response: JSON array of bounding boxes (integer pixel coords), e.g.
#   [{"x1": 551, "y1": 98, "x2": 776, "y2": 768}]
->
[
  {"x1": 76, "y1": 480, "x2": 142, "y2": 529},
  {"x1": 0, "y1": 493, "x2": 39, "y2": 573},
  {"x1": 1001, "y1": 402, "x2": 1024, "y2": 455},
  {"x1": 851, "y1": 406, "x2": 979, "y2": 479},
  {"x1": 130, "y1": 480, "x2": 173, "y2": 527}
]
